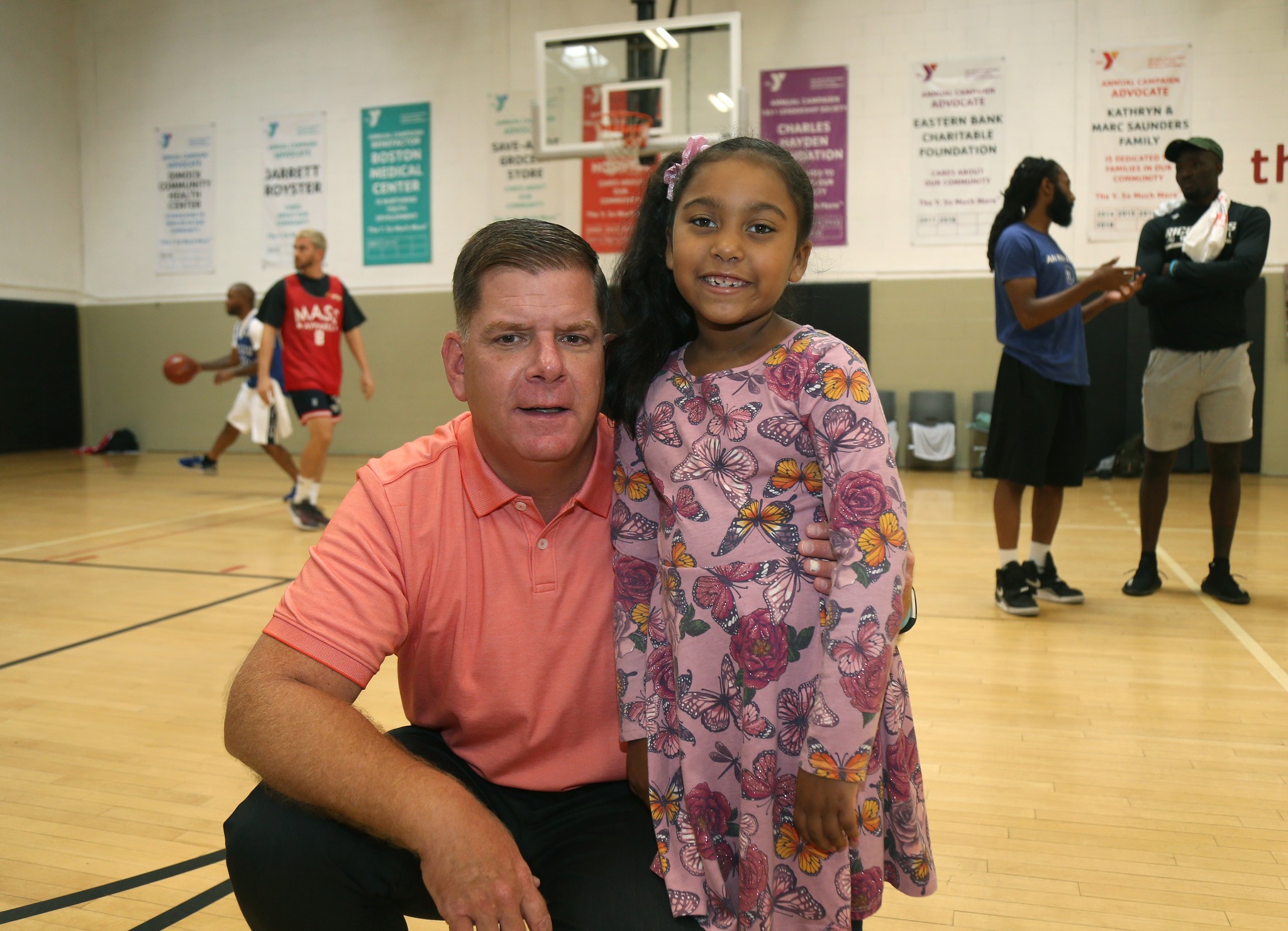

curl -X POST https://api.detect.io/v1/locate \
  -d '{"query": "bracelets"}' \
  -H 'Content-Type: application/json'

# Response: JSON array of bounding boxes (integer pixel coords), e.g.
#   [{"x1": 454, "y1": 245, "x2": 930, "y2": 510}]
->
[{"x1": 897, "y1": 585, "x2": 917, "y2": 634}]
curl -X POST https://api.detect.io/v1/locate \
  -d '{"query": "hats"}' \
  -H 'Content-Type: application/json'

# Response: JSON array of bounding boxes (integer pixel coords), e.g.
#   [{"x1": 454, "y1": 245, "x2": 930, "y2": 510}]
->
[{"x1": 1165, "y1": 137, "x2": 1224, "y2": 163}]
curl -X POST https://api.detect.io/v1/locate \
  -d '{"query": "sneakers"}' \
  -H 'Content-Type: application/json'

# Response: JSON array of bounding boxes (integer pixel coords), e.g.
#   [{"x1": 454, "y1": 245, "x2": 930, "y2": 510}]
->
[
  {"x1": 1121, "y1": 567, "x2": 1167, "y2": 596},
  {"x1": 995, "y1": 561, "x2": 1040, "y2": 616},
  {"x1": 178, "y1": 454, "x2": 218, "y2": 475},
  {"x1": 1201, "y1": 562, "x2": 1250, "y2": 604},
  {"x1": 285, "y1": 487, "x2": 296, "y2": 501},
  {"x1": 1023, "y1": 552, "x2": 1084, "y2": 603},
  {"x1": 290, "y1": 499, "x2": 330, "y2": 529}
]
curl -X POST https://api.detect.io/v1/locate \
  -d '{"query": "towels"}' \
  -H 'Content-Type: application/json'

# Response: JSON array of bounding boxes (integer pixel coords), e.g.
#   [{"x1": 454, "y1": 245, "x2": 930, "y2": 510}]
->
[
  {"x1": 887, "y1": 420, "x2": 901, "y2": 460},
  {"x1": 1155, "y1": 188, "x2": 1232, "y2": 266},
  {"x1": 251, "y1": 377, "x2": 293, "y2": 446},
  {"x1": 908, "y1": 421, "x2": 956, "y2": 461}
]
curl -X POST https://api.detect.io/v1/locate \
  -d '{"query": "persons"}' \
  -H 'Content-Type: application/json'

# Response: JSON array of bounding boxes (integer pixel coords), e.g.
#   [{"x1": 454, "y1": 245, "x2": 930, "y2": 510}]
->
[
  {"x1": 222, "y1": 218, "x2": 702, "y2": 931},
  {"x1": 602, "y1": 136, "x2": 937, "y2": 931},
  {"x1": 253, "y1": 229, "x2": 374, "y2": 530},
  {"x1": 178, "y1": 283, "x2": 298, "y2": 502},
  {"x1": 982, "y1": 155, "x2": 1147, "y2": 618},
  {"x1": 1121, "y1": 137, "x2": 1272, "y2": 605}
]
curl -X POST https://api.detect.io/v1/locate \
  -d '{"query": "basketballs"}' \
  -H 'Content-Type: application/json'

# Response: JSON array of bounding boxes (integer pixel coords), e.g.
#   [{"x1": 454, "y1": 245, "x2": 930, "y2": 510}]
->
[{"x1": 164, "y1": 354, "x2": 195, "y2": 385}]
